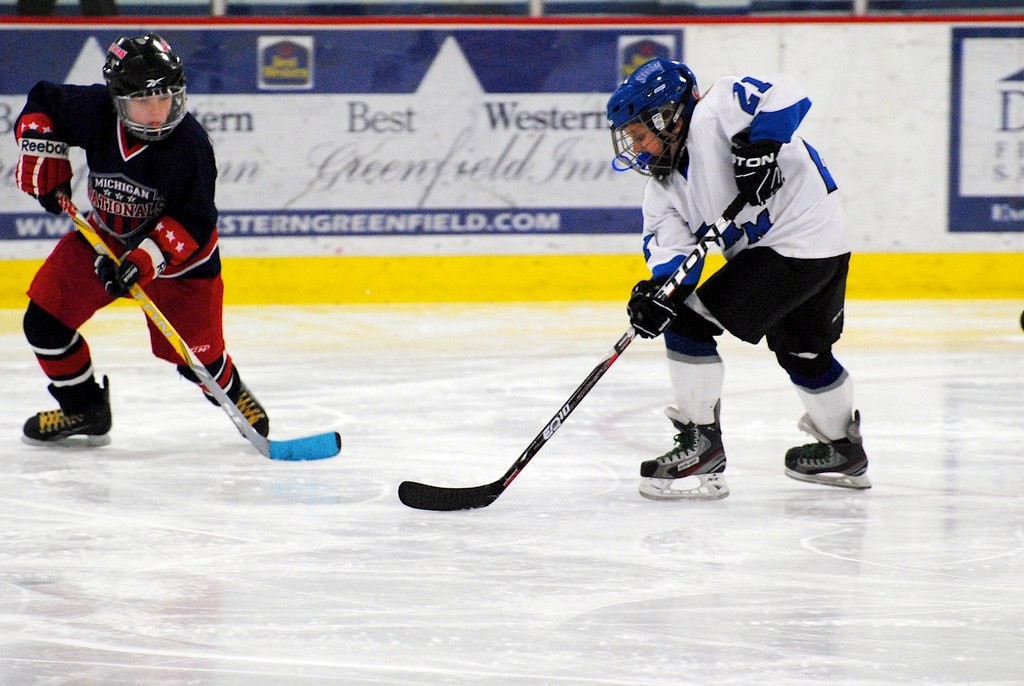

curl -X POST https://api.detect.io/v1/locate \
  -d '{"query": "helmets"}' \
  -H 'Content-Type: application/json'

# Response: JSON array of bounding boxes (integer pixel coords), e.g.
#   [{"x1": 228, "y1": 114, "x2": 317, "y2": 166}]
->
[
  {"x1": 607, "y1": 57, "x2": 700, "y2": 181},
  {"x1": 101, "y1": 33, "x2": 190, "y2": 145}
]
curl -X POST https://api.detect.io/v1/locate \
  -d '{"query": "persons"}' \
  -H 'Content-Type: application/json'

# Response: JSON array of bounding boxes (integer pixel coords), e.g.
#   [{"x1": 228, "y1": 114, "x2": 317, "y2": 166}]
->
[
  {"x1": 15, "y1": 34, "x2": 268, "y2": 449},
  {"x1": 608, "y1": 56, "x2": 872, "y2": 500}
]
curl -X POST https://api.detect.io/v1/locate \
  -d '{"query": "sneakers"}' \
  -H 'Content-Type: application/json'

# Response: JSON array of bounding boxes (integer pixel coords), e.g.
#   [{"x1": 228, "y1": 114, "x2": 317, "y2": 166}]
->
[
  {"x1": 637, "y1": 399, "x2": 728, "y2": 503},
  {"x1": 783, "y1": 408, "x2": 872, "y2": 489},
  {"x1": 204, "y1": 366, "x2": 269, "y2": 440},
  {"x1": 22, "y1": 375, "x2": 113, "y2": 446}
]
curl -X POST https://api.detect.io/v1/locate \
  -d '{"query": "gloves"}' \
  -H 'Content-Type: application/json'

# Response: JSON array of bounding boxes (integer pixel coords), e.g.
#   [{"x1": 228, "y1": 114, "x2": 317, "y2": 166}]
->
[
  {"x1": 94, "y1": 237, "x2": 168, "y2": 298},
  {"x1": 625, "y1": 278, "x2": 679, "y2": 340},
  {"x1": 12, "y1": 132, "x2": 74, "y2": 218},
  {"x1": 728, "y1": 128, "x2": 786, "y2": 207}
]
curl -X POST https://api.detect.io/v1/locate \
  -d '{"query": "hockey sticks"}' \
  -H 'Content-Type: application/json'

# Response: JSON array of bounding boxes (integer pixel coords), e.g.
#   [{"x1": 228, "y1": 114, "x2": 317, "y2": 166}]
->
[
  {"x1": 54, "y1": 189, "x2": 341, "y2": 460},
  {"x1": 397, "y1": 194, "x2": 749, "y2": 512}
]
